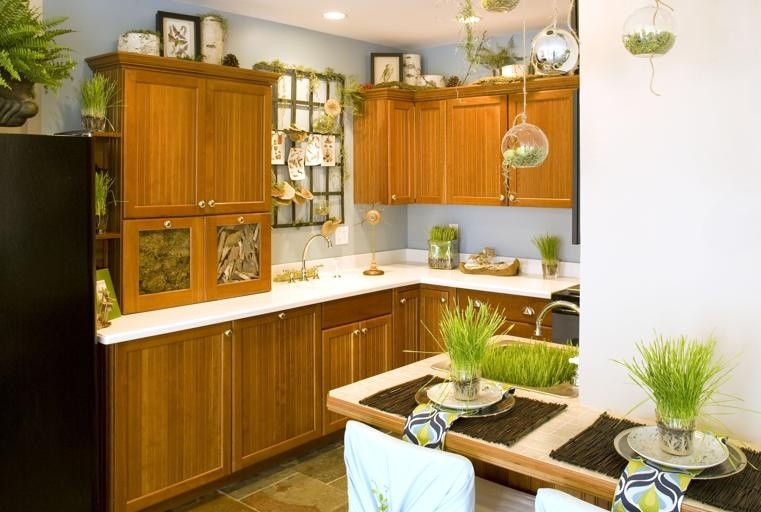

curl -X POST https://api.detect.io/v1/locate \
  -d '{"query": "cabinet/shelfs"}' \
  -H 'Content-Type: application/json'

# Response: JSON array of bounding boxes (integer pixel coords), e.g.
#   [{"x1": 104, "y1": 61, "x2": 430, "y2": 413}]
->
[
  {"x1": 85, "y1": 52, "x2": 281, "y2": 316},
  {"x1": 322, "y1": 283, "x2": 420, "y2": 436},
  {"x1": 353, "y1": 88, "x2": 446, "y2": 206},
  {"x1": 96, "y1": 303, "x2": 321, "y2": 511},
  {"x1": 421, "y1": 285, "x2": 553, "y2": 359},
  {"x1": 446, "y1": 75, "x2": 579, "y2": 208}
]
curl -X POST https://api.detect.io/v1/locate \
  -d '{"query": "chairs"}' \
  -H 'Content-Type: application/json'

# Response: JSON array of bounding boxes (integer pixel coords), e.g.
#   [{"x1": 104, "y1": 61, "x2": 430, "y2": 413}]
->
[
  {"x1": 344, "y1": 417, "x2": 535, "y2": 512},
  {"x1": 535, "y1": 488, "x2": 611, "y2": 512}
]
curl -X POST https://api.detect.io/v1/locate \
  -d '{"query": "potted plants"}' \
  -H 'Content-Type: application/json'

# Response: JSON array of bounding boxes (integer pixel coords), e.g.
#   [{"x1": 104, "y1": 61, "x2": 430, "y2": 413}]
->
[
  {"x1": 0, "y1": 0, "x2": 80, "y2": 127},
  {"x1": 532, "y1": 231, "x2": 559, "y2": 279},
  {"x1": 198, "y1": 10, "x2": 227, "y2": 65},
  {"x1": 117, "y1": 29, "x2": 163, "y2": 57},
  {"x1": 612, "y1": 328, "x2": 761, "y2": 472},
  {"x1": 481, "y1": 0, "x2": 521, "y2": 13},
  {"x1": 501, "y1": 56, "x2": 530, "y2": 78},
  {"x1": 93, "y1": 170, "x2": 130, "y2": 234},
  {"x1": 623, "y1": 0, "x2": 678, "y2": 96},
  {"x1": 80, "y1": 71, "x2": 129, "y2": 132},
  {"x1": 501, "y1": 0, "x2": 549, "y2": 204},
  {"x1": 427, "y1": 223, "x2": 458, "y2": 270},
  {"x1": 401, "y1": 295, "x2": 516, "y2": 417}
]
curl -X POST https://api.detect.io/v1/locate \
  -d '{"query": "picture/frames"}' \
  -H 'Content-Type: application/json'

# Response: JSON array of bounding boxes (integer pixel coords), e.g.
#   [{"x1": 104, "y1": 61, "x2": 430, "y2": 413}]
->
[
  {"x1": 156, "y1": 10, "x2": 201, "y2": 61},
  {"x1": 371, "y1": 52, "x2": 403, "y2": 86}
]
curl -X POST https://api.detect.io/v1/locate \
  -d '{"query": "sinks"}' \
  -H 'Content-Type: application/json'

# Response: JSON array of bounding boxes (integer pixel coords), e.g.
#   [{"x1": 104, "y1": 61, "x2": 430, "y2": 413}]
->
[{"x1": 432, "y1": 339, "x2": 577, "y2": 401}]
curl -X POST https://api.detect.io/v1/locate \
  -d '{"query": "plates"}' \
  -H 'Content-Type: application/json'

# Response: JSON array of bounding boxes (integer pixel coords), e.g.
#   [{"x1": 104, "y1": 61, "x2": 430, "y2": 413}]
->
[
  {"x1": 428, "y1": 381, "x2": 505, "y2": 410},
  {"x1": 630, "y1": 424, "x2": 728, "y2": 467},
  {"x1": 609, "y1": 426, "x2": 749, "y2": 479},
  {"x1": 415, "y1": 380, "x2": 517, "y2": 421}
]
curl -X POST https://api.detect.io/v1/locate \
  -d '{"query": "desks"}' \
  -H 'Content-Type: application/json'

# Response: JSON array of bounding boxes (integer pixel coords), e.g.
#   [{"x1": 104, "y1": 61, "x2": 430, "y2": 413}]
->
[{"x1": 326, "y1": 334, "x2": 761, "y2": 512}]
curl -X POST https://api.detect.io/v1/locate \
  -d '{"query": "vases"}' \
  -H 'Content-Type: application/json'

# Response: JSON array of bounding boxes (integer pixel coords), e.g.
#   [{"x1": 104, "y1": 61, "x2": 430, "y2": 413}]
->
[
  {"x1": 421, "y1": 74, "x2": 445, "y2": 88},
  {"x1": 403, "y1": 54, "x2": 421, "y2": 86}
]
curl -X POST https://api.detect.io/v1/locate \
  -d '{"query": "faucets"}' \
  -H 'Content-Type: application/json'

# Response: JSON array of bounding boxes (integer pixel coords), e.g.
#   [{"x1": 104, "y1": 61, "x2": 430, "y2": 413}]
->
[
  {"x1": 301, "y1": 234, "x2": 331, "y2": 282},
  {"x1": 534, "y1": 300, "x2": 579, "y2": 337}
]
